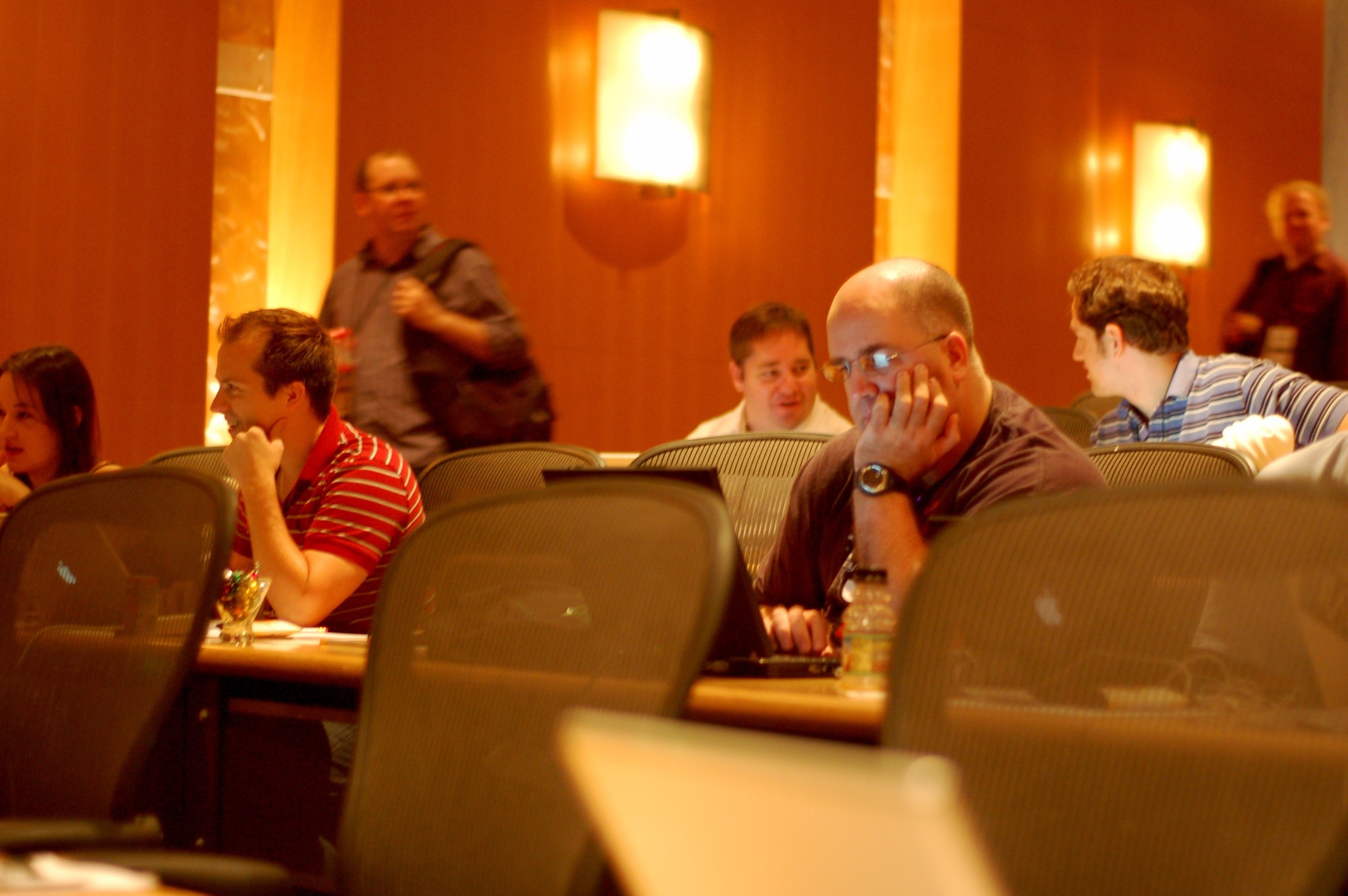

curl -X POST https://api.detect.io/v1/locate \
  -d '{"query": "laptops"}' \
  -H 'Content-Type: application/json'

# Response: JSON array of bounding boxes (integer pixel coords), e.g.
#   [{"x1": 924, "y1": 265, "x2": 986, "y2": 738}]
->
[{"x1": 539, "y1": 465, "x2": 843, "y2": 680}]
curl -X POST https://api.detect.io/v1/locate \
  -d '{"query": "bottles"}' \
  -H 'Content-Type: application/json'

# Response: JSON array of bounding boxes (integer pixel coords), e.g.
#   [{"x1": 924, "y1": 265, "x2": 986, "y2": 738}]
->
[{"x1": 835, "y1": 564, "x2": 897, "y2": 700}]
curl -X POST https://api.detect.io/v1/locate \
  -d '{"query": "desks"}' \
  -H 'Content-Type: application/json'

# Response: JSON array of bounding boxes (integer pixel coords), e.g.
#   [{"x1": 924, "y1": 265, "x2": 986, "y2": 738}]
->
[{"x1": 17, "y1": 614, "x2": 1348, "y2": 849}]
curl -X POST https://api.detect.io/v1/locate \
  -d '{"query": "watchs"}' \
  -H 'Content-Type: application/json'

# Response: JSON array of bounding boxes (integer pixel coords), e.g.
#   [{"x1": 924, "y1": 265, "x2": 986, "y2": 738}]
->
[{"x1": 852, "y1": 461, "x2": 921, "y2": 496}]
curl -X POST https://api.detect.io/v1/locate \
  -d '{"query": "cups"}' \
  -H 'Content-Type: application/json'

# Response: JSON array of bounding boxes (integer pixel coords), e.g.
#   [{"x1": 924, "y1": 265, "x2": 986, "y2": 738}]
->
[{"x1": 216, "y1": 576, "x2": 272, "y2": 647}]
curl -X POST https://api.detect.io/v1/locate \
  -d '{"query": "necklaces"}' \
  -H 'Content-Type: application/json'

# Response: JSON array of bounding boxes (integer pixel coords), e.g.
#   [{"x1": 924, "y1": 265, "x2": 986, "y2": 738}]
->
[{"x1": 341, "y1": 265, "x2": 393, "y2": 337}]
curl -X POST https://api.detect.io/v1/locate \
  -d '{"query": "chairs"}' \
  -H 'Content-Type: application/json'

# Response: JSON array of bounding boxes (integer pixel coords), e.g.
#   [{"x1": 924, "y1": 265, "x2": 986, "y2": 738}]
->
[{"x1": 0, "y1": 379, "x2": 1348, "y2": 896}]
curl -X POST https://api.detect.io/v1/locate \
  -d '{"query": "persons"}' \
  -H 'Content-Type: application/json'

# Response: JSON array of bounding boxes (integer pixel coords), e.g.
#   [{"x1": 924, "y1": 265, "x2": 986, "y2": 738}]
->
[
  {"x1": 0, "y1": 344, "x2": 121, "y2": 523},
  {"x1": 209, "y1": 308, "x2": 429, "y2": 711},
  {"x1": 753, "y1": 258, "x2": 1113, "y2": 655},
  {"x1": 683, "y1": 301, "x2": 855, "y2": 439},
  {"x1": 1066, "y1": 256, "x2": 1348, "y2": 449},
  {"x1": 316, "y1": 148, "x2": 527, "y2": 477},
  {"x1": 1221, "y1": 180, "x2": 1348, "y2": 389}
]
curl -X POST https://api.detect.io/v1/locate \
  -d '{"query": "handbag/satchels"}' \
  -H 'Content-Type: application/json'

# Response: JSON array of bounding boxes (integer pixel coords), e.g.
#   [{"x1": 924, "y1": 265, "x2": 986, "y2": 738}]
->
[{"x1": 398, "y1": 237, "x2": 557, "y2": 450}]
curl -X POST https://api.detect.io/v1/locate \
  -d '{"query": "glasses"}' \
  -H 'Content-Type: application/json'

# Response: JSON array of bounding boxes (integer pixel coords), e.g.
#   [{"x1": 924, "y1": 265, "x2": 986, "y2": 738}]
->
[{"x1": 823, "y1": 334, "x2": 952, "y2": 381}]
